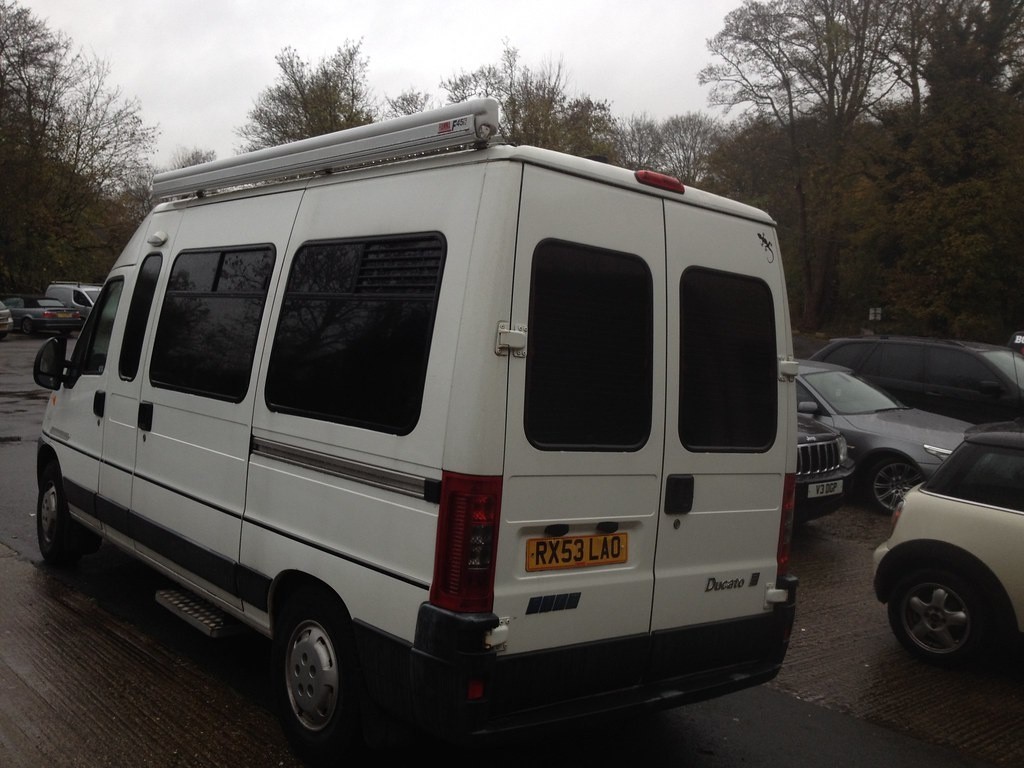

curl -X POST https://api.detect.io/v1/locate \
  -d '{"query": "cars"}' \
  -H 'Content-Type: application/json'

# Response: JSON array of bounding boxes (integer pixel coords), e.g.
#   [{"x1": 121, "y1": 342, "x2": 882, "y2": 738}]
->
[
  {"x1": 0, "y1": 294, "x2": 82, "y2": 335},
  {"x1": 794, "y1": 413, "x2": 858, "y2": 523},
  {"x1": 807, "y1": 335, "x2": 1024, "y2": 425},
  {"x1": 794, "y1": 358, "x2": 979, "y2": 515},
  {"x1": 0, "y1": 301, "x2": 14, "y2": 340},
  {"x1": 872, "y1": 416, "x2": 1023, "y2": 665}
]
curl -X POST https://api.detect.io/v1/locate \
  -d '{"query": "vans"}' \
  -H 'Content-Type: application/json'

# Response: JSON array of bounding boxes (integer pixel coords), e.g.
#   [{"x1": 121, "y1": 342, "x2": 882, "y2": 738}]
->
[
  {"x1": 31, "y1": 100, "x2": 800, "y2": 764},
  {"x1": 45, "y1": 285, "x2": 103, "y2": 328}
]
